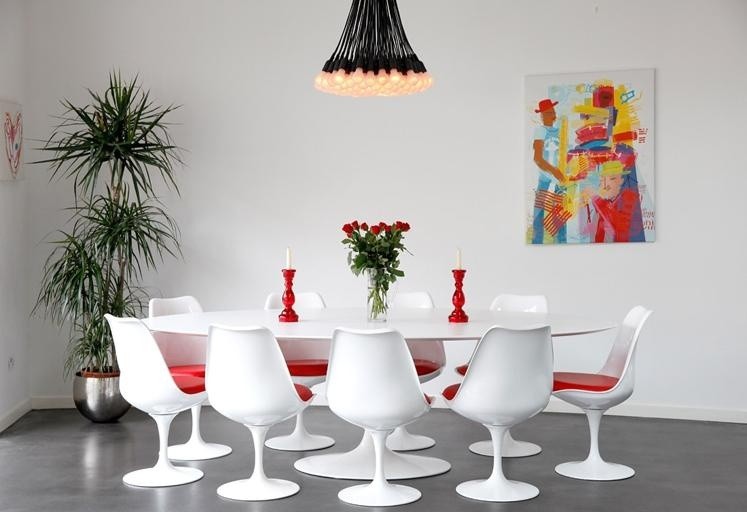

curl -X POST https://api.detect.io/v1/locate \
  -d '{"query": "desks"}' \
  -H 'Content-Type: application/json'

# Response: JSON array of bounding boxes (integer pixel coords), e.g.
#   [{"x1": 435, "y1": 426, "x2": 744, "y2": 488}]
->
[{"x1": 139, "y1": 311, "x2": 618, "y2": 483}]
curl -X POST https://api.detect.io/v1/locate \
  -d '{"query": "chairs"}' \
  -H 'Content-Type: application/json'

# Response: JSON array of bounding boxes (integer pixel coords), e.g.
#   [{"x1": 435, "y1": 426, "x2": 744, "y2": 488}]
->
[
  {"x1": 387, "y1": 293, "x2": 448, "y2": 454},
  {"x1": 324, "y1": 328, "x2": 437, "y2": 505},
  {"x1": 205, "y1": 324, "x2": 317, "y2": 503},
  {"x1": 264, "y1": 292, "x2": 338, "y2": 454},
  {"x1": 102, "y1": 310, "x2": 205, "y2": 491},
  {"x1": 454, "y1": 291, "x2": 551, "y2": 460},
  {"x1": 552, "y1": 303, "x2": 652, "y2": 481},
  {"x1": 441, "y1": 323, "x2": 555, "y2": 505},
  {"x1": 146, "y1": 292, "x2": 233, "y2": 463}
]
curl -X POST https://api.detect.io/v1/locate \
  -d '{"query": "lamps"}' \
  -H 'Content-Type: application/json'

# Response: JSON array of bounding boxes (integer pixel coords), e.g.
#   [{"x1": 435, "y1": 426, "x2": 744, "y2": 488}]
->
[{"x1": 314, "y1": 0, "x2": 434, "y2": 98}]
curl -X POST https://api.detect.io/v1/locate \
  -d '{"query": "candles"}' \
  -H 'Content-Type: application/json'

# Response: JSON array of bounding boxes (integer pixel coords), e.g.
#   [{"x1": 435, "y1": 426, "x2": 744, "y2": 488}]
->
[
  {"x1": 287, "y1": 248, "x2": 292, "y2": 268},
  {"x1": 456, "y1": 250, "x2": 462, "y2": 268}
]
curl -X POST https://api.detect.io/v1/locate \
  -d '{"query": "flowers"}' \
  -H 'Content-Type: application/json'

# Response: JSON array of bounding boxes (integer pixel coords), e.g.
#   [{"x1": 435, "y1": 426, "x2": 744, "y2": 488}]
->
[{"x1": 341, "y1": 219, "x2": 414, "y2": 294}]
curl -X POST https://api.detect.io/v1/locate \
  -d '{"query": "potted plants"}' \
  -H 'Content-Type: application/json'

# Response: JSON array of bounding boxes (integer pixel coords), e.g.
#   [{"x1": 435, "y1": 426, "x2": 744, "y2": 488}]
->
[{"x1": 24, "y1": 69, "x2": 187, "y2": 424}]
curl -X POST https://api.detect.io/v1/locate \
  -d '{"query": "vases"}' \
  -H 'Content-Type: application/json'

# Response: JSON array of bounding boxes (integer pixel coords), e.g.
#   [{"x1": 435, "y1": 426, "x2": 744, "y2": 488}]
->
[{"x1": 365, "y1": 267, "x2": 389, "y2": 321}]
